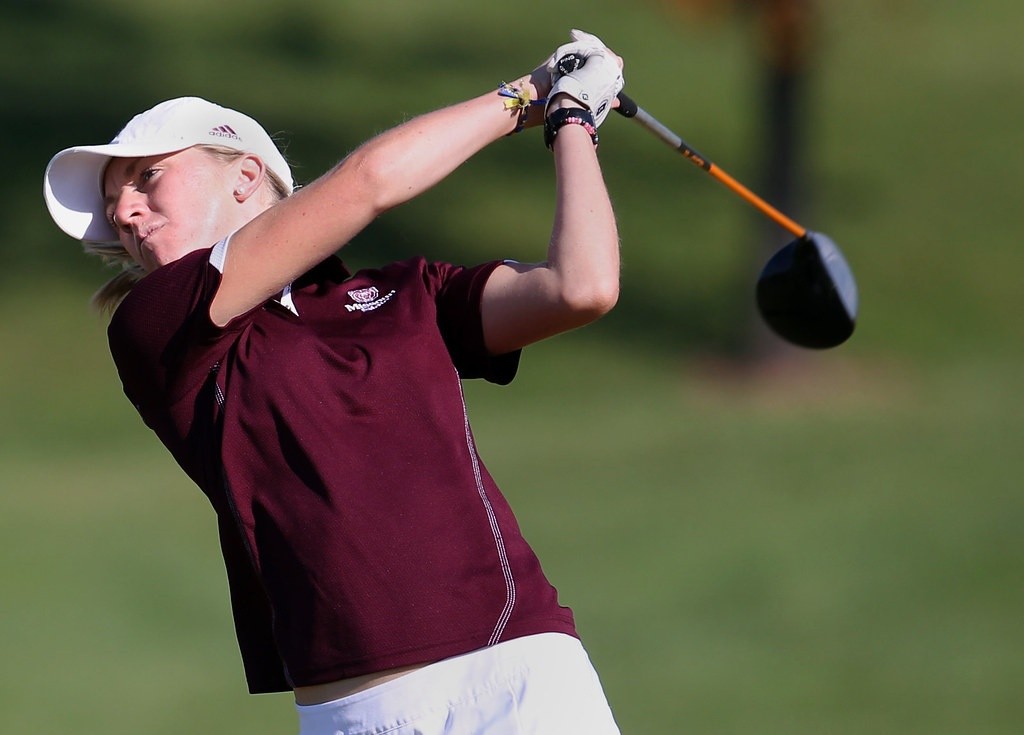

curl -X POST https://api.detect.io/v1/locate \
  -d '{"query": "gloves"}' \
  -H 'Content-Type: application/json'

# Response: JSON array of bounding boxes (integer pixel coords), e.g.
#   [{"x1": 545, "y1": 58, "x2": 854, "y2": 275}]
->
[{"x1": 544, "y1": 29, "x2": 626, "y2": 129}]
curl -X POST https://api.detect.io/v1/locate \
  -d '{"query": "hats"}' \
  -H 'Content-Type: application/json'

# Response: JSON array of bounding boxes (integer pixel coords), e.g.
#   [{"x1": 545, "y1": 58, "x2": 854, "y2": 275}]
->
[{"x1": 43, "y1": 97, "x2": 291, "y2": 243}]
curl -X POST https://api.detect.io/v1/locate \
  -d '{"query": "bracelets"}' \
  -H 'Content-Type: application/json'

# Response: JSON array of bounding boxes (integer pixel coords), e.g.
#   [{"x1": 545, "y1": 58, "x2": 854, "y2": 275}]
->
[
  {"x1": 543, "y1": 107, "x2": 598, "y2": 156},
  {"x1": 498, "y1": 77, "x2": 531, "y2": 137}
]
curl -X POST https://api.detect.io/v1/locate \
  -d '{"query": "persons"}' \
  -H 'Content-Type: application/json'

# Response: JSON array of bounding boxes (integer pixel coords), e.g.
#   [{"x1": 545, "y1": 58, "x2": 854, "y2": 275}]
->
[{"x1": 38, "y1": 26, "x2": 620, "y2": 735}]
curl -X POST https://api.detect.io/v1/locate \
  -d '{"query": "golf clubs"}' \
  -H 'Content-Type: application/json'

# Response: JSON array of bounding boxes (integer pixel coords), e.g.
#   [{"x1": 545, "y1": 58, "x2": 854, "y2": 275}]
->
[{"x1": 557, "y1": 52, "x2": 860, "y2": 351}]
